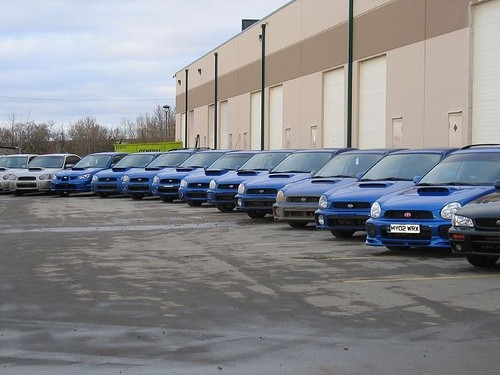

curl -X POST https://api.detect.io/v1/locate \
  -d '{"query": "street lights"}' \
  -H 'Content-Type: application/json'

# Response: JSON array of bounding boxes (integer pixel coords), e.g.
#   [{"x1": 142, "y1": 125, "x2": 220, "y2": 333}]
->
[{"x1": 163, "y1": 104, "x2": 170, "y2": 142}]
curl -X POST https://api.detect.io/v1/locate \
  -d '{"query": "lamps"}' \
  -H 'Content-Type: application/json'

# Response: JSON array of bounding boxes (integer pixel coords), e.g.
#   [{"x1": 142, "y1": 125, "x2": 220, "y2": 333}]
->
[
  {"x1": 259, "y1": 35, "x2": 262, "y2": 41},
  {"x1": 178, "y1": 80, "x2": 181, "y2": 85},
  {"x1": 198, "y1": 68, "x2": 201, "y2": 75}
]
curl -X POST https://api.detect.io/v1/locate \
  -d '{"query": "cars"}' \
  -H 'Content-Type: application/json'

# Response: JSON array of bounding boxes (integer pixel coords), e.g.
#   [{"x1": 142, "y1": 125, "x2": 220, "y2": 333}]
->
[{"x1": 0, "y1": 143, "x2": 500, "y2": 270}]
